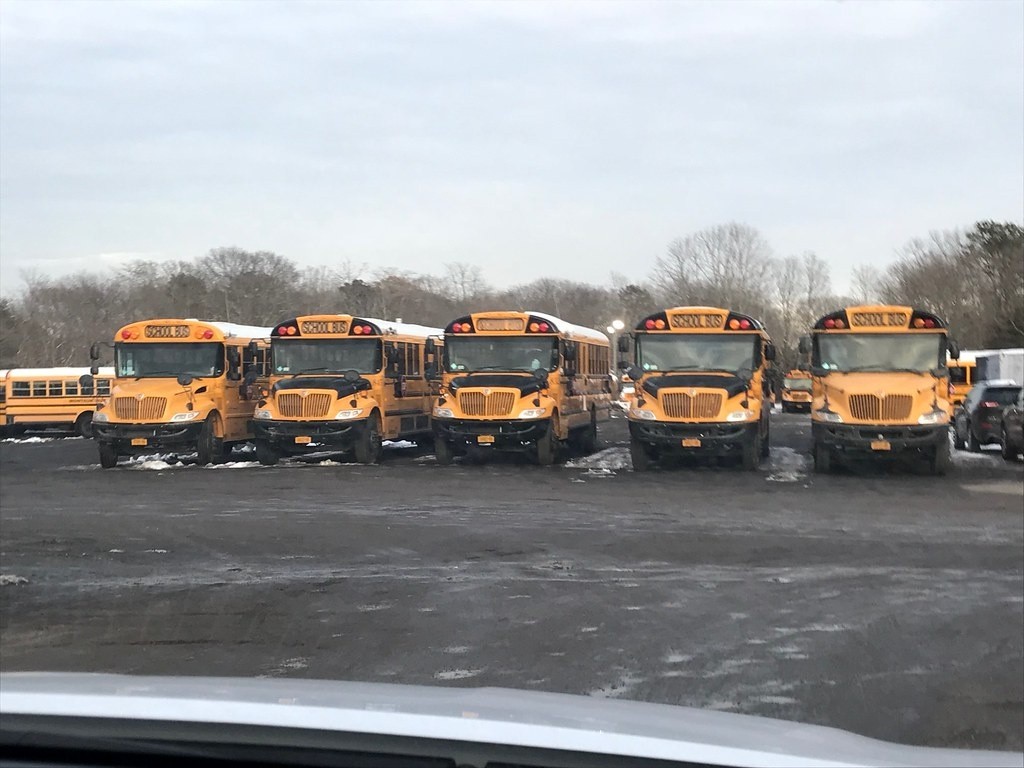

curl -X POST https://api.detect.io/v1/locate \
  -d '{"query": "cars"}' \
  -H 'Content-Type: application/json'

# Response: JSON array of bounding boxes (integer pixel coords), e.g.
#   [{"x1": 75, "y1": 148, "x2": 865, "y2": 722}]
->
[{"x1": 1000, "y1": 388, "x2": 1024, "y2": 460}]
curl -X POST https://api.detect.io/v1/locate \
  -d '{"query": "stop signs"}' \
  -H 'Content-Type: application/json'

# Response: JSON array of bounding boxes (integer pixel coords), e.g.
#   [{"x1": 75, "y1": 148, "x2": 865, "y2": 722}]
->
[
  {"x1": 245, "y1": 383, "x2": 253, "y2": 400},
  {"x1": 400, "y1": 374, "x2": 407, "y2": 395},
  {"x1": 571, "y1": 378, "x2": 577, "y2": 395}
]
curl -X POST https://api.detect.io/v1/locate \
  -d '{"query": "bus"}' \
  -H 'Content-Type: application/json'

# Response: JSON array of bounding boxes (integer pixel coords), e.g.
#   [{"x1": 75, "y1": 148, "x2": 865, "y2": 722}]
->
[
  {"x1": 0, "y1": 366, "x2": 129, "y2": 438},
  {"x1": 780, "y1": 370, "x2": 812, "y2": 414},
  {"x1": 423, "y1": 310, "x2": 611, "y2": 465},
  {"x1": 244, "y1": 313, "x2": 472, "y2": 467},
  {"x1": 80, "y1": 317, "x2": 318, "y2": 468},
  {"x1": 944, "y1": 349, "x2": 978, "y2": 424},
  {"x1": 618, "y1": 374, "x2": 637, "y2": 411},
  {"x1": 798, "y1": 305, "x2": 960, "y2": 475},
  {"x1": 616, "y1": 306, "x2": 776, "y2": 472}
]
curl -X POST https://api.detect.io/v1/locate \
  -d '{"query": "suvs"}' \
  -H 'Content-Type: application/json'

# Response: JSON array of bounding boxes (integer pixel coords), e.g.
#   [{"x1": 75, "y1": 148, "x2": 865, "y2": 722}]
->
[{"x1": 952, "y1": 380, "x2": 1022, "y2": 452}]
